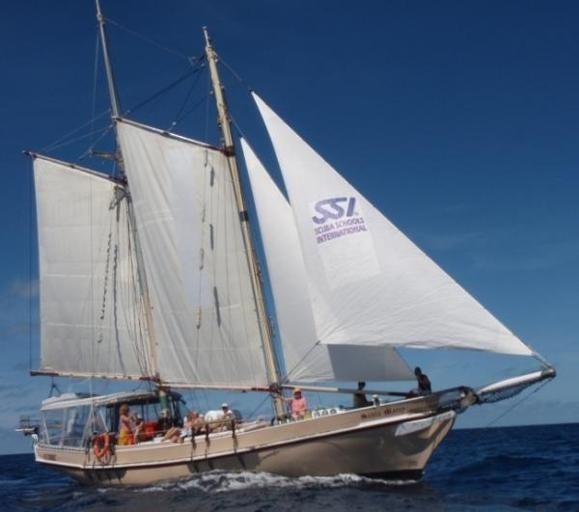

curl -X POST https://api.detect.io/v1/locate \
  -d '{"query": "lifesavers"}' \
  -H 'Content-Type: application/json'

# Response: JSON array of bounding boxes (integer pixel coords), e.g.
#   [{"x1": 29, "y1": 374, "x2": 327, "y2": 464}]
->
[{"x1": 93, "y1": 432, "x2": 110, "y2": 458}]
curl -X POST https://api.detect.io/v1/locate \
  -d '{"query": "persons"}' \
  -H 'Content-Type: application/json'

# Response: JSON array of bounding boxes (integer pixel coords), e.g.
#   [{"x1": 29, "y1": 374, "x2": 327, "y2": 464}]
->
[
  {"x1": 117, "y1": 404, "x2": 137, "y2": 445},
  {"x1": 162, "y1": 403, "x2": 236, "y2": 441},
  {"x1": 406, "y1": 367, "x2": 431, "y2": 398},
  {"x1": 282, "y1": 388, "x2": 307, "y2": 418},
  {"x1": 353, "y1": 380, "x2": 373, "y2": 408}
]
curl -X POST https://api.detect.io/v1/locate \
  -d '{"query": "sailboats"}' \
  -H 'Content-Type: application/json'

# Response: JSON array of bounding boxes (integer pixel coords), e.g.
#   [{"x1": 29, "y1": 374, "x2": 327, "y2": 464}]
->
[{"x1": 14, "y1": 1, "x2": 555, "y2": 490}]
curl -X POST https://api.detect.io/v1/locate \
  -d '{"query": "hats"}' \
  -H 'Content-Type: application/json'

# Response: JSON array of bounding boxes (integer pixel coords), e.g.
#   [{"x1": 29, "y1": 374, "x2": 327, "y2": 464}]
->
[
  {"x1": 222, "y1": 402, "x2": 228, "y2": 408},
  {"x1": 293, "y1": 388, "x2": 301, "y2": 393}
]
